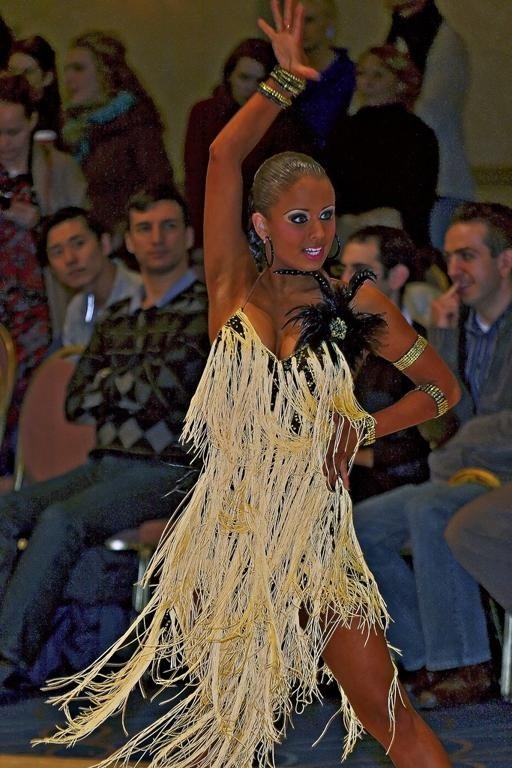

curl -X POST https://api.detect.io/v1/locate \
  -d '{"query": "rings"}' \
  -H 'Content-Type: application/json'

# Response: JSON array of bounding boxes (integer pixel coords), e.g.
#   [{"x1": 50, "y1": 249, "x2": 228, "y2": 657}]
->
[{"x1": 282, "y1": 21, "x2": 292, "y2": 29}]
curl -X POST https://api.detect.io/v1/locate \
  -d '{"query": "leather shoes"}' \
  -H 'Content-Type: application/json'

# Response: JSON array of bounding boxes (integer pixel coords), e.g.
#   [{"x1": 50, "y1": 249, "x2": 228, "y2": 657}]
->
[{"x1": 418, "y1": 663, "x2": 498, "y2": 709}]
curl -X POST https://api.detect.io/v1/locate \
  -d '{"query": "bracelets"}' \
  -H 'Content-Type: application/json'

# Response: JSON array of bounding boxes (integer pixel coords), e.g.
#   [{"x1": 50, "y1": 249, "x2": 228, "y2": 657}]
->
[
  {"x1": 414, "y1": 383, "x2": 449, "y2": 421},
  {"x1": 258, "y1": 78, "x2": 296, "y2": 108},
  {"x1": 366, "y1": 414, "x2": 379, "y2": 445},
  {"x1": 269, "y1": 70, "x2": 308, "y2": 96}
]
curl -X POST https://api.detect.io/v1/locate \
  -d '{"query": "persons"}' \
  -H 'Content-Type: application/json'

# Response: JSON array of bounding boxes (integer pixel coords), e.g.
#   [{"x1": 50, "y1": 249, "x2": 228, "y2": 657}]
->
[
  {"x1": 2, "y1": 186, "x2": 213, "y2": 703},
  {"x1": 32, "y1": 2, "x2": 461, "y2": 767},
  {"x1": 328, "y1": 1, "x2": 473, "y2": 242},
  {"x1": 0, "y1": 20, "x2": 174, "y2": 225},
  {"x1": 291, "y1": 1, "x2": 361, "y2": 151},
  {"x1": 338, "y1": 225, "x2": 428, "y2": 502},
  {"x1": 352, "y1": 203, "x2": 512, "y2": 712},
  {"x1": 183, "y1": 35, "x2": 299, "y2": 249},
  {"x1": 42, "y1": 205, "x2": 146, "y2": 363},
  {"x1": 442, "y1": 482, "x2": 512, "y2": 621},
  {"x1": 3, "y1": 162, "x2": 53, "y2": 465}
]
